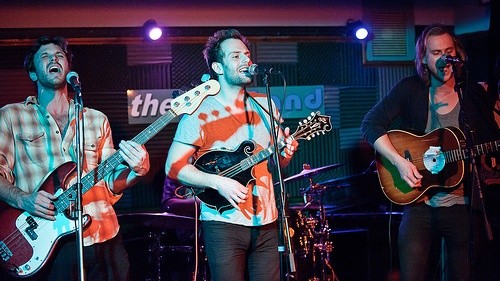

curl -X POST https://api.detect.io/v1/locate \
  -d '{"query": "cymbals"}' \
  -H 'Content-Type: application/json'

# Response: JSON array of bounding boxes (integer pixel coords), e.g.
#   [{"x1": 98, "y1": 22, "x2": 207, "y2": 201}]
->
[
  {"x1": 117, "y1": 210, "x2": 200, "y2": 229},
  {"x1": 273, "y1": 162, "x2": 341, "y2": 186}
]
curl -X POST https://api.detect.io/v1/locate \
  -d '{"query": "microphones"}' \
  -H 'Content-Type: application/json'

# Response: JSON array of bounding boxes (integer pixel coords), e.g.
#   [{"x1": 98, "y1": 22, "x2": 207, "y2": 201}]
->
[
  {"x1": 249, "y1": 64, "x2": 280, "y2": 76},
  {"x1": 441, "y1": 54, "x2": 464, "y2": 63},
  {"x1": 66, "y1": 72, "x2": 81, "y2": 97}
]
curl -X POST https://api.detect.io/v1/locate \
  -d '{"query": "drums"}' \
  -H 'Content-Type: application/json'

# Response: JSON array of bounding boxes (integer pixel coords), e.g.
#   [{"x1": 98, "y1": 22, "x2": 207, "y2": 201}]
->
[{"x1": 283, "y1": 211, "x2": 336, "y2": 281}]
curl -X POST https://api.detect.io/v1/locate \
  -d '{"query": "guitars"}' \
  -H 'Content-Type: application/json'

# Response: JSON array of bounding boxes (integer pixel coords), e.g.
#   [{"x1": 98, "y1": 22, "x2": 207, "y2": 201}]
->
[
  {"x1": 374, "y1": 126, "x2": 500, "y2": 206},
  {"x1": 0, "y1": 73, "x2": 220, "y2": 281},
  {"x1": 188, "y1": 110, "x2": 333, "y2": 212}
]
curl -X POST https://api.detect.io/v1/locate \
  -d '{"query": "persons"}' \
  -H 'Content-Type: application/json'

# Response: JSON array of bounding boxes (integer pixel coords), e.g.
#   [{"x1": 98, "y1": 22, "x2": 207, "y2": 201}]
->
[
  {"x1": 161, "y1": 176, "x2": 201, "y2": 241},
  {"x1": 166, "y1": 27, "x2": 299, "y2": 281},
  {"x1": 0, "y1": 35, "x2": 151, "y2": 281},
  {"x1": 361, "y1": 23, "x2": 500, "y2": 281}
]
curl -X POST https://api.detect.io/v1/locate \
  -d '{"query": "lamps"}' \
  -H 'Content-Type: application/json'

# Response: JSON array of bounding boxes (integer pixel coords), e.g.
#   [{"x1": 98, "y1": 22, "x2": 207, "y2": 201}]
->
[
  {"x1": 143, "y1": 21, "x2": 162, "y2": 41},
  {"x1": 348, "y1": 18, "x2": 370, "y2": 41}
]
